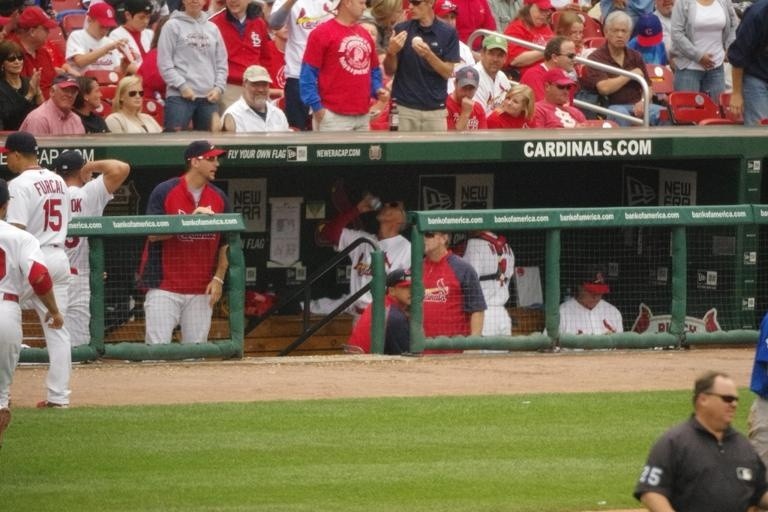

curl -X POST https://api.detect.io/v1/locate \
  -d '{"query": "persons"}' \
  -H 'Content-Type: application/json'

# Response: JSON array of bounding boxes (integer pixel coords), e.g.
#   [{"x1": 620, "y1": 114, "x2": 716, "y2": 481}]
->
[
  {"x1": 65, "y1": 2, "x2": 136, "y2": 83},
  {"x1": 533, "y1": 68, "x2": 588, "y2": 127},
  {"x1": 54, "y1": 151, "x2": 131, "y2": 348},
  {"x1": 134, "y1": 141, "x2": 233, "y2": 344},
  {"x1": 521, "y1": 39, "x2": 577, "y2": 103},
  {"x1": 423, "y1": 232, "x2": 487, "y2": 355},
  {"x1": 266, "y1": 21, "x2": 290, "y2": 102},
  {"x1": 581, "y1": 10, "x2": 667, "y2": 128},
  {"x1": 373, "y1": 0, "x2": 404, "y2": 28},
  {"x1": 356, "y1": 21, "x2": 383, "y2": 46},
  {"x1": 629, "y1": 17, "x2": 673, "y2": 67},
  {"x1": 434, "y1": 2, "x2": 476, "y2": 95},
  {"x1": 268, "y1": 0, "x2": 338, "y2": 131},
  {"x1": 552, "y1": 12, "x2": 586, "y2": 59},
  {"x1": 436, "y1": 1, "x2": 497, "y2": 50},
  {"x1": 654, "y1": 2, "x2": 675, "y2": 48},
  {"x1": 105, "y1": 76, "x2": 236, "y2": 133},
  {"x1": 471, "y1": 33, "x2": 511, "y2": 113},
  {"x1": 72, "y1": 76, "x2": 111, "y2": 133},
  {"x1": 543, "y1": 272, "x2": 623, "y2": 349},
  {"x1": 14, "y1": 5, "x2": 58, "y2": 100},
  {"x1": 670, "y1": 0, "x2": 738, "y2": 104},
  {"x1": 16, "y1": 74, "x2": 85, "y2": 137},
  {"x1": 446, "y1": 67, "x2": 487, "y2": 130},
  {"x1": 0, "y1": 0, "x2": 54, "y2": 17},
  {"x1": 300, "y1": 0, "x2": 390, "y2": 131},
  {"x1": 633, "y1": 370, "x2": 768, "y2": 512},
  {"x1": 321, "y1": 193, "x2": 412, "y2": 329},
  {"x1": 504, "y1": 0, "x2": 558, "y2": 72},
  {"x1": 209, "y1": 1, "x2": 273, "y2": 87},
  {"x1": 349, "y1": 271, "x2": 413, "y2": 353},
  {"x1": 0, "y1": 179, "x2": 64, "y2": 436},
  {"x1": 486, "y1": 85, "x2": 535, "y2": 129},
  {"x1": 0, "y1": 41, "x2": 45, "y2": 131},
  {"x1": 748, "y1": 315, "x2": 768, "y2": 465},
  {"x1": 111, "y1": 1, "x2": 156, "y2": 72},
  {"x1": 221, "y1": 65, "x2": 293, "y2": 131},
  {"x1": 4, "y1": 133, "x2": 72, "y2": 410},
  {"x1": 725, "y1": 1, "x2": 767, "y2": 125},
  {"x1": 147, "y1": 15, "x2": 164, "y2": 32},
  {"x1": 156, "y1": 1, "x2": 228, "y2": 133},
  {"x1": 383, "y1": 1, "x2": 460, "y2": 131},
  {"x1": 601, "y1": 0, "x2": 656, "y2": 23},
  {"x1": 459, "y1": 230, "x2": 516, "y2": 355}
]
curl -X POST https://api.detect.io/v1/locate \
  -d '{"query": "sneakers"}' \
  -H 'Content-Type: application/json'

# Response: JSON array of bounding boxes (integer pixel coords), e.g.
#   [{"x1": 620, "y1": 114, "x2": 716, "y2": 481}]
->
[
  {"x1": 38, "y1": 400, "x2": 69, "y2": 409},
  {"x1": 0, "y1": 408, "x2": 12, "y2": 438}
]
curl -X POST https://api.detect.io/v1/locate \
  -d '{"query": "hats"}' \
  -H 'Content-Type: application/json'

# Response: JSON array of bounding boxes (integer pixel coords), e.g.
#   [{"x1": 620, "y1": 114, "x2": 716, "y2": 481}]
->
[
  {"x1": 483, "y1": 34, "x2": 508, "y2": 53},
  {"x1": 53, "y1": 73, "x2": 82, "y2": 90},
  {"x1": 433, "y1": 1, "x2": 461, "y2": 16},
  {"x1": 545, "y1": 69, "x2": 576, "y2": 86},
  {"x1": 577, "y1": 270, "x2": 610, "y2": 293},
  {"x1": 19, "y1": 6, "x2": 60, "y2": 29},
  {"x1": 0, "y1": 179, "x2": 15, "y2": 206},
  {"x1": 55, "y1": 151, "x2": 87, "y2": 173},
  {"x1": 88, "y1": 4, "x2": 118, "y2": 27},
  {"x1": 243, "y1": 65, "x2": 273, "y2": 83},
  {"x1": 184, "y1": 140, "x2": 224, "y2": 164},
  {"x1": 638, "y1": 14, "x2": 664, "y2": 46},
  {"x1": 387, "y1": 269, "x2": 413, "y2": 288},
  {"x1": 525, "y1": 0, "x2": 556, "y2": 11},
  {"x1": 328, "y1": 0, "x2": 341, "y2": 11},
  {"x1": 0, "y1": 132, "x2": 37, "y2": 153},
  {"x1": 455, "y1": 66, "x2": 480, "y2": 89}
]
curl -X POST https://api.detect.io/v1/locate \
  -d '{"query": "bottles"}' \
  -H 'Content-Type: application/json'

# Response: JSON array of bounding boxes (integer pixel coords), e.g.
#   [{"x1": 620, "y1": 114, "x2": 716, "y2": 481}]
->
[
  {"x1": 390, "y1": 104, "x2": 398, "y2": 131},
  {"x1": 360, "y1": 189, "x2": 385, "y2": 213},
  {"x1": 392, "y1": 98, "x2": 397, "y2": 105}
]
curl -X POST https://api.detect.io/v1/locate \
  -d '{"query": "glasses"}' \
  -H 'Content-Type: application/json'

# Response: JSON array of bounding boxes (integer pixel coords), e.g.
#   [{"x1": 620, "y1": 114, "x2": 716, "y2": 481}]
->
[
  {"x1": 561, "y1": 52, "x2": 576, "y2": 57},
  {"x1": 6, "y1": 55, "x2": 25, "y2": 62},
  {"x1": 389, "y1": 201, "x2": 405, "y2": 217},
  {"x1": 408, "y1": 0, "x2": 427, "y2": 6},
  {"x1": 204, "y1": 156, "x2": 218, "y2": 162},
  {"x1": 127, "y1": 90, "x2": 144, "y2": 96},
  {"x1": 425, "y1": 231, "x2": 444, "y2": 239},
  {"x1": 554, "y1": 84, "x2": 573, "y2": 89},
  {"x1": 706, "y1": 391, "x2": 740, "y2": 404}
]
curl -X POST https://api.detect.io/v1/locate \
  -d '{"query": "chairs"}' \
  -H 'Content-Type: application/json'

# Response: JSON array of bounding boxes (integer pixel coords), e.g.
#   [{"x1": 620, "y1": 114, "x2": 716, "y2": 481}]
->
[
  {"x1": 582, "y1": 36, "x2": 607, "y2": 49},
  {"x1": 718, "y1": 93, "x2": 733, "y2": 116},
  {"x1": 51, "y1": 0, "x2": 84, "y2": 15},
  {"x1": 63, "y1": 14, "x2": 85, "y2": 37},
  {"x1": 551, "y1": 9, "x2": 602, "y2": 39},
  {"x1": 644, "y1": 62, "x2": 675, "y2": 98},
  {"x1": 667, "y1": 91, "x2": 722, "y2": 125}
]
